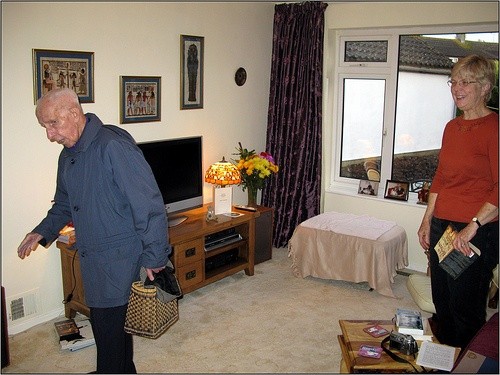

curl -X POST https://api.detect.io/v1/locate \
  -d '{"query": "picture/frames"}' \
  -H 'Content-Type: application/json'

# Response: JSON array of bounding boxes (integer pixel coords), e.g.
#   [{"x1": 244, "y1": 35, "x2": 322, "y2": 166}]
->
[
  {"x1": 179, "y1": 35, "x2": 205, "y2": 110},
  {"x1": 358, "y1": 180, "x2": 379, "y2": 195},
  {"x1": 382, "y1": 179, "x2": 409, "y2": 201},
  {"x1": 119, "y1": 76, "x2": 163, "y2": 124},
  {"x1": 32, "y1": 48, "x2": 96, "y2": 104}
]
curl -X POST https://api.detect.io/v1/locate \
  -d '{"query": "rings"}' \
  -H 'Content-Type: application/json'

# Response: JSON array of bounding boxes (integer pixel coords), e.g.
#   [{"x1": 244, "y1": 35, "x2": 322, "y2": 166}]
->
[{"x1": 461, "y1": 243, "x2": 465, "y2": 247}]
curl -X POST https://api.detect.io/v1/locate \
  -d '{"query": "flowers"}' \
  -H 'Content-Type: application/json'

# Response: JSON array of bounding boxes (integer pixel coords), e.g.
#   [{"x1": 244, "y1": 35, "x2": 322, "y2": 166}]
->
[{"x1": 230, "y1": 140, "x2": 280, "y2": 188}]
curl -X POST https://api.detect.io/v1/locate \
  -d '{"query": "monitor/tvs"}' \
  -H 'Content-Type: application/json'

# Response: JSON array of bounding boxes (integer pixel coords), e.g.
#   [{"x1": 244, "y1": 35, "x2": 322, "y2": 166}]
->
[{"x1": 136, "y1": 135, "x2": 204, "y2": 227}]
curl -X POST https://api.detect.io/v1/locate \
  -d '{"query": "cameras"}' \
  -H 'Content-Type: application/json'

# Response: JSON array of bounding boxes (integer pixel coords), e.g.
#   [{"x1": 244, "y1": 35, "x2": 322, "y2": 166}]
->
[{"x1": 390, "y1": 331, "x2": 418, "y2": 356}]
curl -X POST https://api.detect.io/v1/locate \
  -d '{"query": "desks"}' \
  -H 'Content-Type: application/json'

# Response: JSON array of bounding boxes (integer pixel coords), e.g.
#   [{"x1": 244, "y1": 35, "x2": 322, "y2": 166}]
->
[
  {"x1": 289, "y1": 212, "x2": 409, "y2": 299},
  {"x1": 337, "y1": 318, "x2": 458, "y2": 374}
]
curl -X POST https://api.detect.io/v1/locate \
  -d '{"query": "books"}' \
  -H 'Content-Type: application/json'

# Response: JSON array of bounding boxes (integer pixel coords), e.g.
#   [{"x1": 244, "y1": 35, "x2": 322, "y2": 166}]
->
[
  {"x1": 396, "y1": 310, "x2": 423, "y2": 335},
  {"x1": 53, "y1": 319, "x2": 96, "y2": 352},
  {"x1": 412, "y1": 318, "x2": 433, "y2": 342},
  {"x1": 434, "y1": 222, "x2": 481, "y2": 280}
]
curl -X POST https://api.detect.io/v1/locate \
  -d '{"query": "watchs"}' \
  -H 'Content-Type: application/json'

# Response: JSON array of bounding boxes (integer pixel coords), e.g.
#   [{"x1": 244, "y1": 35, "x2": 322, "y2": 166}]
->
[{"x1": 472, "y1": 217, "x2": 481, "y2": 228}]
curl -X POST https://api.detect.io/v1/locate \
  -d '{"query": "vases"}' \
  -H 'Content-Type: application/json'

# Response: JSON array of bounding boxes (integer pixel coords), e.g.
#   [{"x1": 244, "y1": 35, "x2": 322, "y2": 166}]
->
[{"x1": 247, "y1": 182, "x2": 259, "y2": 207}]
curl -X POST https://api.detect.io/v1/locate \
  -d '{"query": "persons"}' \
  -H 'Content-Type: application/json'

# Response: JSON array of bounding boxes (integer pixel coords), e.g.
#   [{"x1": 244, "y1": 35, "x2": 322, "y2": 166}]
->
[
  {"x1": 17, "y1": 89, "x2": 171, "y2": 374},
  {"x1": 417, "y1": 55, "x2": 499, "y2": 349},
  {"x1": 419, "y1": 182, "x2": 430, "y2": 202},
  {"x1": 391, "y1": 184, "x2": 405, "y2": 196}
]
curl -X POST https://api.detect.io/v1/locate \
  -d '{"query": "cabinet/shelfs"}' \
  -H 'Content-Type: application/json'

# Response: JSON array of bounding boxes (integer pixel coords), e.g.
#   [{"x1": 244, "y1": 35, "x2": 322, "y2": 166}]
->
[
  {"x1": 56, "y1": 204, "x2": 256, "y2": 322},
  {"x1": 235, "y1": 204, "x2": 274, "y2": 265}
]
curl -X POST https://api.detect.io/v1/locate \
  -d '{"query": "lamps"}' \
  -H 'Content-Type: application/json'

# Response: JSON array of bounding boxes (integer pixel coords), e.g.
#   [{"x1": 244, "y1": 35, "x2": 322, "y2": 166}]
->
[{"x1": 205, "y1": 156, "x2": 241, "y2": 214}]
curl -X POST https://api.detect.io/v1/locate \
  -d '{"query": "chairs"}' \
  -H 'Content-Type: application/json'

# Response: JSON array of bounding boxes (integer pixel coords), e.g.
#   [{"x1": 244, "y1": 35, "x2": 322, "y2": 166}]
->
[
  {"x1": 408, "y1": 251, "x2": 439, "y2": 314},
  {"x1": 365, "y1": 159, "x2": 432, "y2": 194}
]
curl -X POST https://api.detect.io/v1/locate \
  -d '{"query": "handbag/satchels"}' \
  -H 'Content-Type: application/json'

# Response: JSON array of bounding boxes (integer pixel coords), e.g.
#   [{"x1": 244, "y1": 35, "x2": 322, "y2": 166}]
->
[{"x1": 123, "y1": 280, "x2": 179, "y2": 340}]
